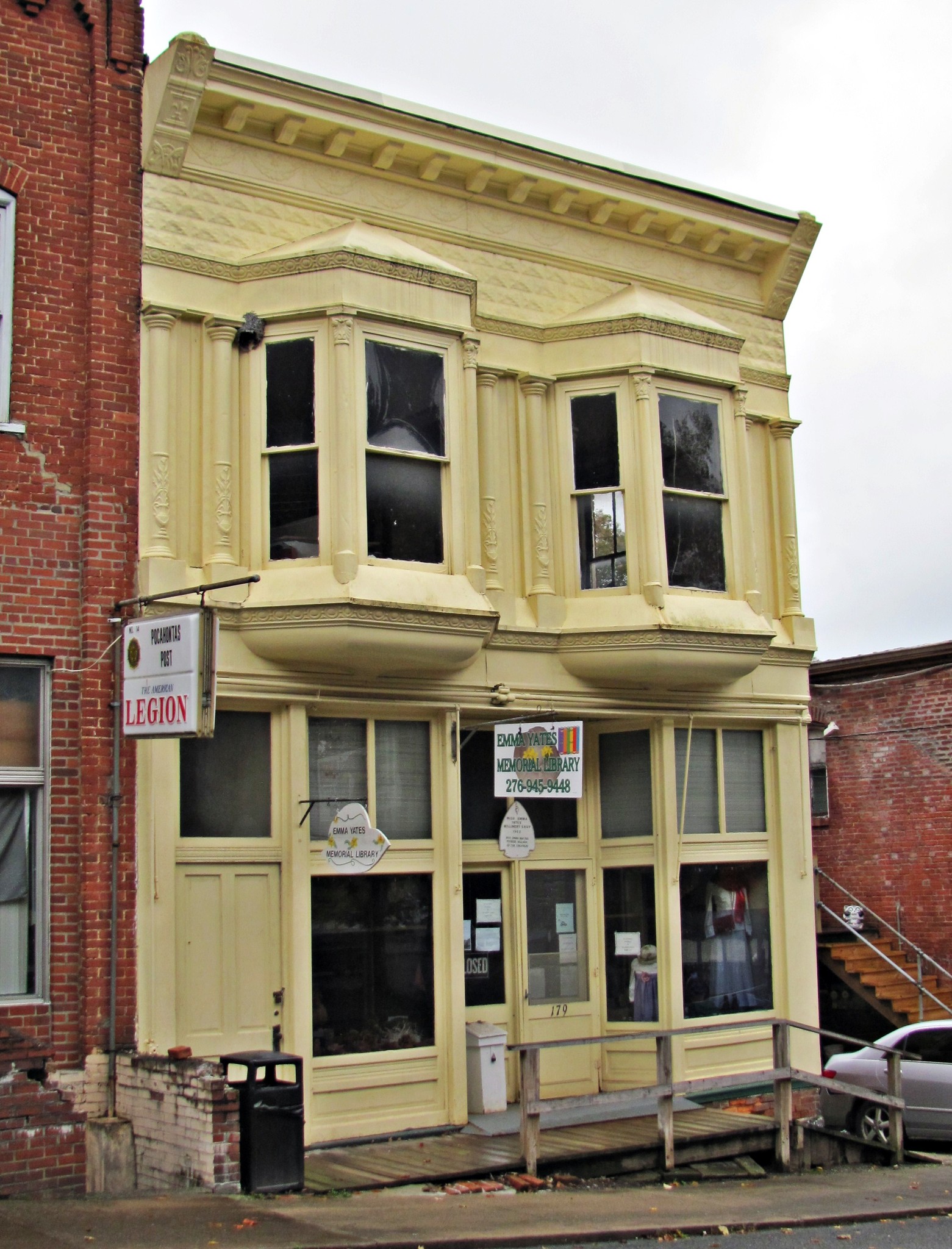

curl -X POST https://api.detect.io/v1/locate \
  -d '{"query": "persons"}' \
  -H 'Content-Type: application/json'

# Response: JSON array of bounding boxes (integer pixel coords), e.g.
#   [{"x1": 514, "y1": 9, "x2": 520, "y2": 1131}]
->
[
  {"x1": 704, "y1": 868, "x2": 761, "y2": 1008},
  {"x1": 627, "y1": 944, "x2": 656, "y2": 1021}
]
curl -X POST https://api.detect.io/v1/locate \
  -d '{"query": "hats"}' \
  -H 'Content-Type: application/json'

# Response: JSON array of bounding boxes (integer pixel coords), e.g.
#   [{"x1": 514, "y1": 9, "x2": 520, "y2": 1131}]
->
[{"x1": 637, "y1": 944, "x2": 659, "y2": 964}]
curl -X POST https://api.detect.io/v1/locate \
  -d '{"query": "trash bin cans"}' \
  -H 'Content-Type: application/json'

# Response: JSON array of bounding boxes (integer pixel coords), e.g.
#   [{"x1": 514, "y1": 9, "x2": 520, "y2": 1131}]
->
[
  {"x1": 468, "y1": 1023, "x2": 509, "y2": 1117},
  {"x1": 219, "y1": 1052, "x2": 305, "y2": 1195}
]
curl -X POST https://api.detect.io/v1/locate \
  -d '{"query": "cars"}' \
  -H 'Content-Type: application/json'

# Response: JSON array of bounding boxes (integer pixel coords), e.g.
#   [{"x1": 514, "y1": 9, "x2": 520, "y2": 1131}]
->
[{"x1": 820, "y1": 1019, "x2": 952, "y2": 1167}]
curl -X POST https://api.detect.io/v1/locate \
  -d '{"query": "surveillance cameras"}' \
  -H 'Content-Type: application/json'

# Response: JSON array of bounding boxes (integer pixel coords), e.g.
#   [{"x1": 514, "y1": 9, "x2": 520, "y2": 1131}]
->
[{"x1": 823, "y1": 724, "x2": 840, "y2": 736}]
